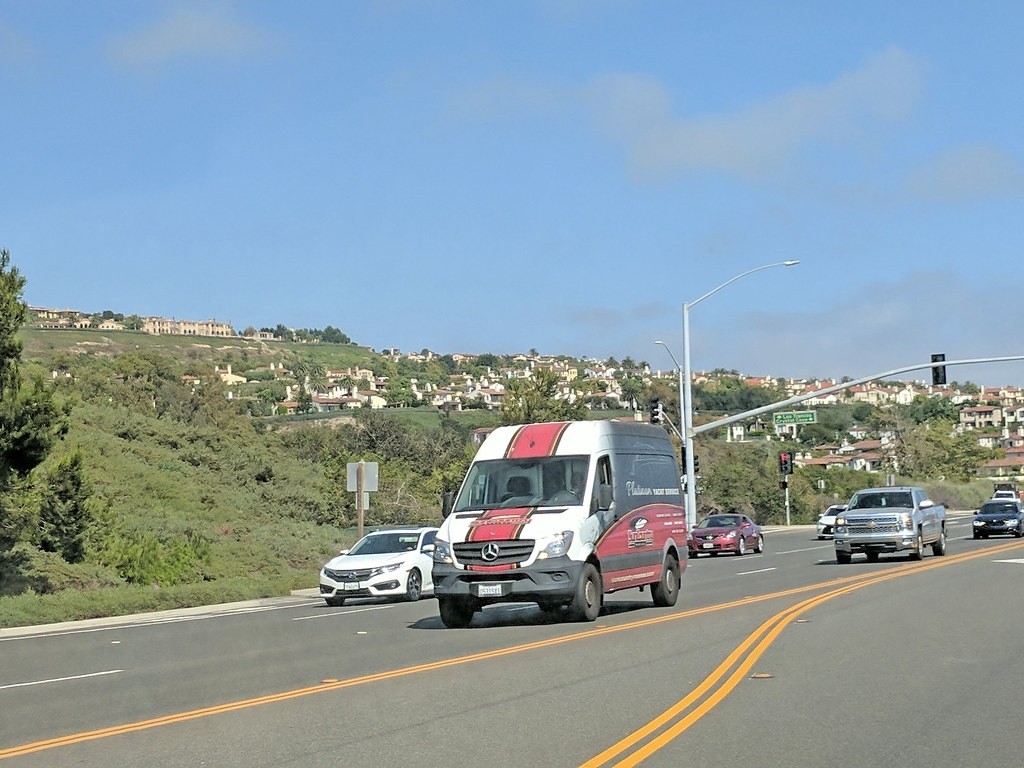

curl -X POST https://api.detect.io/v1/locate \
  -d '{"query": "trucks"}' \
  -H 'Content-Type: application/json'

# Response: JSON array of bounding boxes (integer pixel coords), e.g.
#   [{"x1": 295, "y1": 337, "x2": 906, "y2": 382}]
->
[{"x1": 430, "y1": 420, "x2": 689, "y2": 628}]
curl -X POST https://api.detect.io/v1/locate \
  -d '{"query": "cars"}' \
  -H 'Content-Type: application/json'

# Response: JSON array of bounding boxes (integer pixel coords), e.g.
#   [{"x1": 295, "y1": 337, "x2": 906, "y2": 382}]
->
[
  {"x1": 816, "y1": 505, "x2": 849, "y2": 540},
  {"x1": 973, "y1": 491, "x2": 1024, "y2": 538},
  {"x1": 318, "y1": 527, "x2": 441, "y2": 608},
  {"x1": 687, "y1": 513, "x2": 763, "y2": 557}
]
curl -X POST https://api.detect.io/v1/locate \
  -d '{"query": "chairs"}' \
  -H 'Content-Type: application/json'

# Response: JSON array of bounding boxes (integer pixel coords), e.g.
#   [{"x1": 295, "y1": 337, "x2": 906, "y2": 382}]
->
[{"x1": 500, "y1": 476, "x2": 534, "y2": 503}]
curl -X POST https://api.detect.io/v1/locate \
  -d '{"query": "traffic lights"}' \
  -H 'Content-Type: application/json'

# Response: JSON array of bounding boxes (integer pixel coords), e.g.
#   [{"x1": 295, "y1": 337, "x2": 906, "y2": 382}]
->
[{"x1": 780, "y1": 453, "x2": 791, "y2": 474}]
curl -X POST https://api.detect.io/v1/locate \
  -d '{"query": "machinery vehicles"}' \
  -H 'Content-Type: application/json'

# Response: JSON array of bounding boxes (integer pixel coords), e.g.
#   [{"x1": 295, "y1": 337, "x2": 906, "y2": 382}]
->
[{"x1": 994, "y1": 482, "x2": 1024, "y2": 499}]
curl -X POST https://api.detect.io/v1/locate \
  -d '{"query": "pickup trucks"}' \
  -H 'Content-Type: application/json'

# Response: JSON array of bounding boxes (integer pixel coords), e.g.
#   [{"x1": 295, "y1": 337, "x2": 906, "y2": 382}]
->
[{"x1": 833, "y1": 486, "x2": 949, "y2": 563}]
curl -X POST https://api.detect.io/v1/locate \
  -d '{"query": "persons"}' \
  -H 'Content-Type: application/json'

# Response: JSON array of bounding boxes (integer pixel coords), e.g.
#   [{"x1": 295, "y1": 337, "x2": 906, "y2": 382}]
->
[{"x1": 571, "y1": 472, "x2": 585, "y2": 497}]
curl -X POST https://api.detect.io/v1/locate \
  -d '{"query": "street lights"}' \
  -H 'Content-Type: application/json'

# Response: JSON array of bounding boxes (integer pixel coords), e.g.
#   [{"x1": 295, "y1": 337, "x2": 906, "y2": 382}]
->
[
  {"x1": 653, "y1": 342, "x2": 696, "y2": 537},
  {"x1": 682, "y1": 260, "x2": 801, "y2": 537}
]
